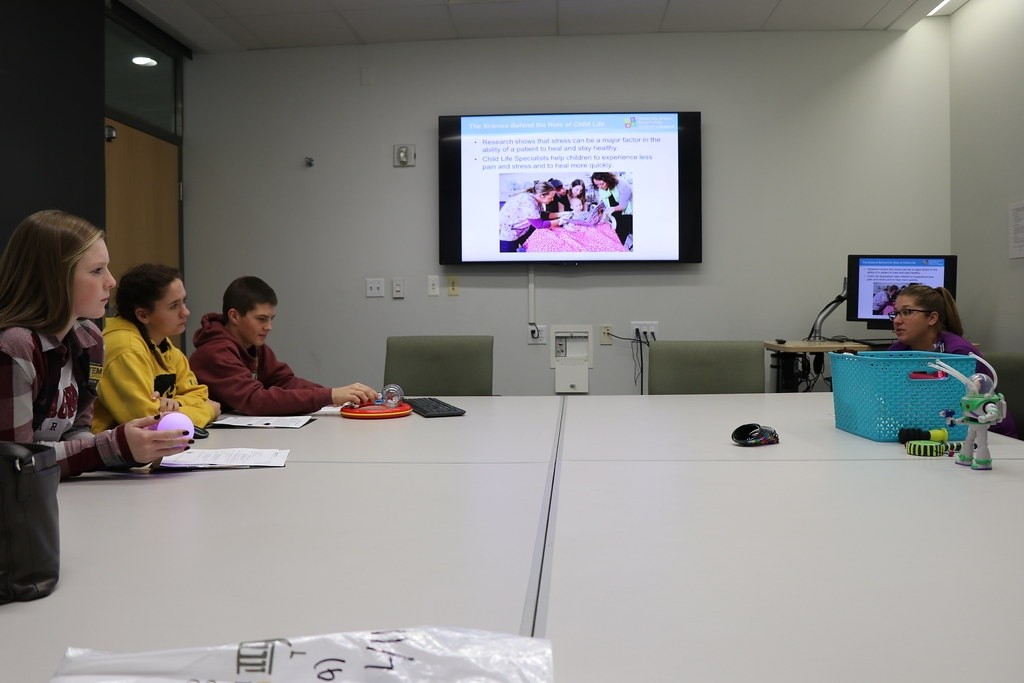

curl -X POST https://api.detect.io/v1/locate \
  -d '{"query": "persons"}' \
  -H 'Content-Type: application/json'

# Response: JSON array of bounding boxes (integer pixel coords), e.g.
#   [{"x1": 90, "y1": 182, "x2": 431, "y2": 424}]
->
[
  {"x1": 0, "y1": 210, "x2": 196, "y2": 483},
  {"x1": 882, "y1": 286, "x2": 1017, "y2": 437},
  {"x1": 946, "y1": 373, "x2": 1000, "y2": 471},
  {"x1": 872, "y1": 283, "x2": 924, "y2": 317},
  {"x1": 90, "y1": 263, "x2": 223, "y2": 470},
  {"x1": 497, "y1": 172, "x2": 634, "y2": 252},
  {"x1": 188, "y1": 276, "x2": 381, "y2": 416}
]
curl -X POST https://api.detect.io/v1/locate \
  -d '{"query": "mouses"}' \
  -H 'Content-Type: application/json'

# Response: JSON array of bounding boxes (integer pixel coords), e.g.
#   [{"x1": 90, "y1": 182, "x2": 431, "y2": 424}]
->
[{"x1": 193, "y1": 425, "x2": 209, "y2": 439}]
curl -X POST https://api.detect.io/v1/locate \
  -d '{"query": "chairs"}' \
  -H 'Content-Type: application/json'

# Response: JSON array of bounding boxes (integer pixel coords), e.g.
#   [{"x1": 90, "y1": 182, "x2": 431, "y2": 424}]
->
[
  {"x1": 384, "y1": 334, "x2": 493, "y2": 398},
  {"x1": 647, "y1": 339, "x2": 768, "y2": 395}
]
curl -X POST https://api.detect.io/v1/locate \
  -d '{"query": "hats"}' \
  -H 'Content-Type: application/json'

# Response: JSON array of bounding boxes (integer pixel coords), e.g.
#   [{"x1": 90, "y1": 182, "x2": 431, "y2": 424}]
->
[{"x1": 552, "y1": 179, "x2": 563, "y2": 188}]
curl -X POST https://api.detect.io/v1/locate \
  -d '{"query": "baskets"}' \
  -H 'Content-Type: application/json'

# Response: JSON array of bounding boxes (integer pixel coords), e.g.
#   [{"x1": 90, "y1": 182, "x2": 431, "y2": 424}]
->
[{"x1": 829, "y1": 350, "x2": 998, "y2": 442}]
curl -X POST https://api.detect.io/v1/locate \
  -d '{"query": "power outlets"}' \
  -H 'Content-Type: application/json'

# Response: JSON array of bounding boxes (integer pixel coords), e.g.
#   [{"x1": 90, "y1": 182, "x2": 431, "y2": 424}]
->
[
  {"x1": 366, "y1": 279, "x2": 385, "y2": 298},
  {"x1": 630, "y1": 322, "x2": 659, "y2": 343},
  {"x1": 448, "y1": 275, "x2": 460, "y2": 296},
  {"x1": 599, "y1": 325, "x2": 613, "y2": 346},
  {"x1": 428, "y1": 275, "x2": 439, "y2": 297},
  {"x1": 528, "y1": 325, "x2": 547, "y2": 346},
  {"x1": 393, "y1": 144, "x2": 416, "y2": 168}
]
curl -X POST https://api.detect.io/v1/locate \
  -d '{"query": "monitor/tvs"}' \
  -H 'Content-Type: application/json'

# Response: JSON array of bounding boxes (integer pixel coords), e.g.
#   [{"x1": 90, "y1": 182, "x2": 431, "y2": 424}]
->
[
  {"x1": 438, "y1": 111, "x2": 702, "y2": 265},
  {"x1": 845, "y1": 255, "x2": 957, "y2": 322}
]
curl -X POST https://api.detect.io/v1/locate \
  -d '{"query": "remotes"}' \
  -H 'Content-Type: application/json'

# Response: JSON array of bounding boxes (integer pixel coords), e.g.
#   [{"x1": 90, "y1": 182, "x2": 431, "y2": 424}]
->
[{"x1": 775, "y1": 339, "x2": 786, "y2": 344}]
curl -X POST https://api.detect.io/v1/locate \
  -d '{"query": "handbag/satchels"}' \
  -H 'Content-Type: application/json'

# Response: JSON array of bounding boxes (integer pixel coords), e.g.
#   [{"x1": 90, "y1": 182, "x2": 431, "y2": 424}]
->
[
  {"x1": 50, "y1": 627, "x2": 552, "y2": 683},
  {"x1": 0, "y1": 441, "x2": 60, "y2": 605}
]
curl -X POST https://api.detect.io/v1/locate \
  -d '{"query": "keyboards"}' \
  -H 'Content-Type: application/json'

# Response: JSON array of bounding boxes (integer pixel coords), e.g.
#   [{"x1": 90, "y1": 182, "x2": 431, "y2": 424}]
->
[{"x1": 403, "y1": 397, "x2": 466, "y2": 418}]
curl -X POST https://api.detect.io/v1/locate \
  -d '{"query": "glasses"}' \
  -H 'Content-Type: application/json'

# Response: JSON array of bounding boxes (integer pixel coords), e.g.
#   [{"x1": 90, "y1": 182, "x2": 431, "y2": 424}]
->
[{"x1": 888, "y1": 309, "x2": 931, "y2": 321}]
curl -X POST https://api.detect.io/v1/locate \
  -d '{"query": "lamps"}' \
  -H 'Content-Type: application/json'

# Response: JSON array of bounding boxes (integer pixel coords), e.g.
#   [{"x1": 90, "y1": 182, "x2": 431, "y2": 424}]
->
[{"x1": 131, "y1": 42, "x2": 160, "y2": 67}]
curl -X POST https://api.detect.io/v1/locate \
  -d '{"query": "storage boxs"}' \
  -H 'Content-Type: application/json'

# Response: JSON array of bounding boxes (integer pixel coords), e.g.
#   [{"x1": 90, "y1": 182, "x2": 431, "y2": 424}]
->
[{"x1": 830, "y1": 349, "x2": 977, "y2": 446}]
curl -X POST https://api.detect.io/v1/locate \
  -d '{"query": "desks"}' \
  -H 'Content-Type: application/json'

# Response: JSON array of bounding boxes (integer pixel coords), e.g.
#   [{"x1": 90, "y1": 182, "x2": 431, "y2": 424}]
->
[
  {"x1": 766, "y1": 341, "x2": 981, "y2": 393},
  {"x1": 0, "y1": 394, "x2": 1024, "y2": 683}
]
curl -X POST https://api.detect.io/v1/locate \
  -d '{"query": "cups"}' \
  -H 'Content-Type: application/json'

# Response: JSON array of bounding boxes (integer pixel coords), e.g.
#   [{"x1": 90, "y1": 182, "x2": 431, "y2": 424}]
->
[{"x1": 382, "y1": 384, "x2": 404, "y2": 407}]
